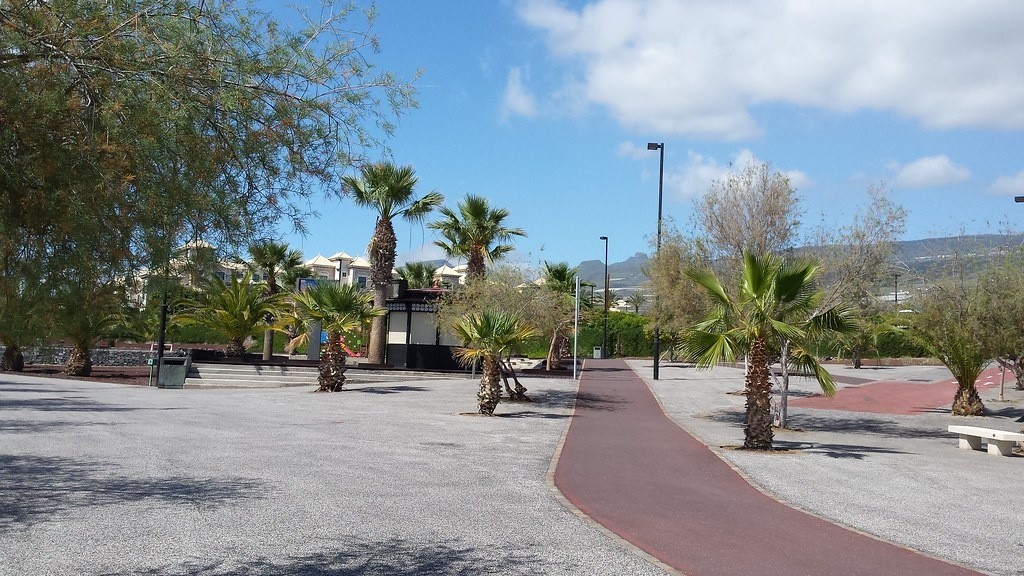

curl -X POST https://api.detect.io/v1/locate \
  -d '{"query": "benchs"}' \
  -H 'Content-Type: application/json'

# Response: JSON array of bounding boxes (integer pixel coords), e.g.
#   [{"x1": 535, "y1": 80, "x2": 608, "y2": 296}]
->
[{"x1": 948, "y1": 425, "x2": 1023, "y2": 456}]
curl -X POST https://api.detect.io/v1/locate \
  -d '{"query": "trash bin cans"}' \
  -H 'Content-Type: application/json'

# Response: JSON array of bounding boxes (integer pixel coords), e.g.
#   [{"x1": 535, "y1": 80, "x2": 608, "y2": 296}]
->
[
  {"x1": 593, "y1": 346, "x2": 601, "y2": 359},
  {"x1": 157, "y1": 357, "x2": 190, "y2": 390}
]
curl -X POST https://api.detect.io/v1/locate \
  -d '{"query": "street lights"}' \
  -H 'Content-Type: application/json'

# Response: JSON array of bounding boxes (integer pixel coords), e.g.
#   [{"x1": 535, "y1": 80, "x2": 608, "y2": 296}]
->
[
  {"x1": 600, "y1": 236, "x2": 608, "y2": 359},
  {"x1": 647, "y1": 141, "x2": 664, "y2": 379},
  {"x1": 336, "y1": 256, "x2": 343, "y2": 289},
  {"x1": 891, "y1": 274, "x2": 901, "y2": 308}
]
